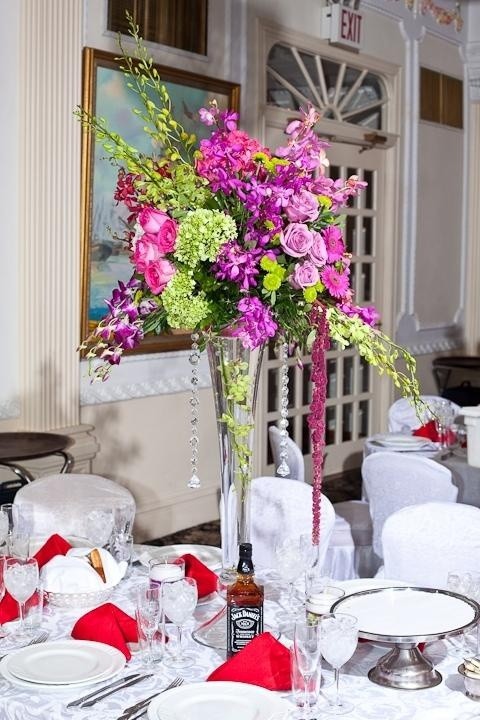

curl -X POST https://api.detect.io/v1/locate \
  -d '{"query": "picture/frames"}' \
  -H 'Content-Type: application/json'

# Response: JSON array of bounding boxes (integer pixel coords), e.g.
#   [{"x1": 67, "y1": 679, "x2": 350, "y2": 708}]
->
[{"x1": 78, "y1": 42, "x2": 244, "y2": 362}]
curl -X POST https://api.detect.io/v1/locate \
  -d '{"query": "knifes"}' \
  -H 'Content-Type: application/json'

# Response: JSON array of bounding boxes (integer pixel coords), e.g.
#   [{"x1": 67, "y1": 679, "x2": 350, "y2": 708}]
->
[{"x1": 69, "y1": 673, "x2": 155, "y2": 708}]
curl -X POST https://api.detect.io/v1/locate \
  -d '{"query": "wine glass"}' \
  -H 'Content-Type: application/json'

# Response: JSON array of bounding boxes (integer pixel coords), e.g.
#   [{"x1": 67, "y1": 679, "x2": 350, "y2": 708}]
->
[
  {"x1": 274, "y1": 533, "x2": 359, "y2": 720},
  {"x1": 0, "y1": 496, "x2": 198, "y2": 668},
  {"x1": 447, "y1": 569, "x2": 480, "y2": 653},
  {"x1": 434, "y1": 399, "x2": 455, "y2": 450}
]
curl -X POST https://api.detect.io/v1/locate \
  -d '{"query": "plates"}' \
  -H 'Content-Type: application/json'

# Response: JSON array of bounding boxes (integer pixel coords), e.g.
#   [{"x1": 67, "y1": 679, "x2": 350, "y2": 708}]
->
[
  {"x1": 0, "y1": 640, "x2": 127, "y2": 690},
  {"x1": 374, "y1": 435, "x2": 431, "y2": 452},
  {"x1": 138, "y1": 544, "x2": 224, "y2": 571},
  {"x1": 147, "y1": 680, "x2": 287, "y2": 720}
]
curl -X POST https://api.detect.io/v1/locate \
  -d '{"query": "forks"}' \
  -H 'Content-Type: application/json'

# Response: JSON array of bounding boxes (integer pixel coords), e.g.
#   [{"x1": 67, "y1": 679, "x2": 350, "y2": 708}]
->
[{"x1": 123, "y1": 677, "x2": 185, "y2": 714}]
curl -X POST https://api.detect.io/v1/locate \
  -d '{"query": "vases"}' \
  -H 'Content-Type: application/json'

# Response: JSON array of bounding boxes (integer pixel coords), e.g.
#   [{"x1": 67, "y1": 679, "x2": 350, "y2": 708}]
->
[{"x1": 201, "y1": 336, "x2": 270, "y2": 577}]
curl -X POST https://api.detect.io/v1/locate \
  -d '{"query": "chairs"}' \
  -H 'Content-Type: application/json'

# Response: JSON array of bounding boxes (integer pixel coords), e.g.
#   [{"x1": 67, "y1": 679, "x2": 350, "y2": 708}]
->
[
  {"x1": 232, "y1": 476, "x2": 336, "y2": 582},
  {"x1": 381, "y1": 503, "x2": 480, "y2": 587},
  {"x1": 388, "y1": 396, "x2": 461, "y2": 436},
  {"x1": 361, "y1": 451, "x2": 458, "y2": 557},
  {"x1": 12, "y1": 473, "x2": 137, "y2": 548},
  {"x1": 269, "y1": 426, "x2": 358, "y2": 580}
]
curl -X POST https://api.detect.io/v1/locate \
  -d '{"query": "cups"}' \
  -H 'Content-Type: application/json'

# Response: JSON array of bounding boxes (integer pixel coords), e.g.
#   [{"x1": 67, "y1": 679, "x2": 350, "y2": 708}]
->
[{"x1": 459, "y1": 407, "x2": 480, "y2": 468}]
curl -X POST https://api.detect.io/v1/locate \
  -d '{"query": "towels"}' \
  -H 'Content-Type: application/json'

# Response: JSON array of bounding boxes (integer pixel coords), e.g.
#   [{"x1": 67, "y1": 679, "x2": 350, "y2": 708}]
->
[
  {"x1": 0, "y1": 555, "x2": 50, "y2": 625},
  {"x1": 206, "y1": 632, "x2": 321, "y2": 692},
  {"x1": 30, "y1": 533, "x2": 73, "y2": 570},
  {"x1": 174, "y1": 553, "x2": 221, "y2": 598},
  {"x1": 71, "y1": 602, "x2": 163, "y2": 663},
  {"x1": 413, "y1": 420, "x2": 457, "y2": 446}
]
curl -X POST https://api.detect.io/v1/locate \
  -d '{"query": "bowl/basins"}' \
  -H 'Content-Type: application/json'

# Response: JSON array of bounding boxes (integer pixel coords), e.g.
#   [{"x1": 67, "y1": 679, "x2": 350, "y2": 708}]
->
[{"x1": 458, "y1": 660, "x2": 480, "y2": 698}]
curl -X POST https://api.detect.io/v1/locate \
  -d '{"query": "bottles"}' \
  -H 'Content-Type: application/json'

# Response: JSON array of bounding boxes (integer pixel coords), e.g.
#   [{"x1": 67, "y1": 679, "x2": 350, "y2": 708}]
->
[{"x1": 228, "y1": 543, "x2": 264, "y2": 659}]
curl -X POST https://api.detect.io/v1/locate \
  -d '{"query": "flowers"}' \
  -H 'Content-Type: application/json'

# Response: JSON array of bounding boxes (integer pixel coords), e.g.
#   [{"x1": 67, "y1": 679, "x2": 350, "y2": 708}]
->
[{"x1": 53, "y1": 7, "x2": 452, "y2": 447}]
